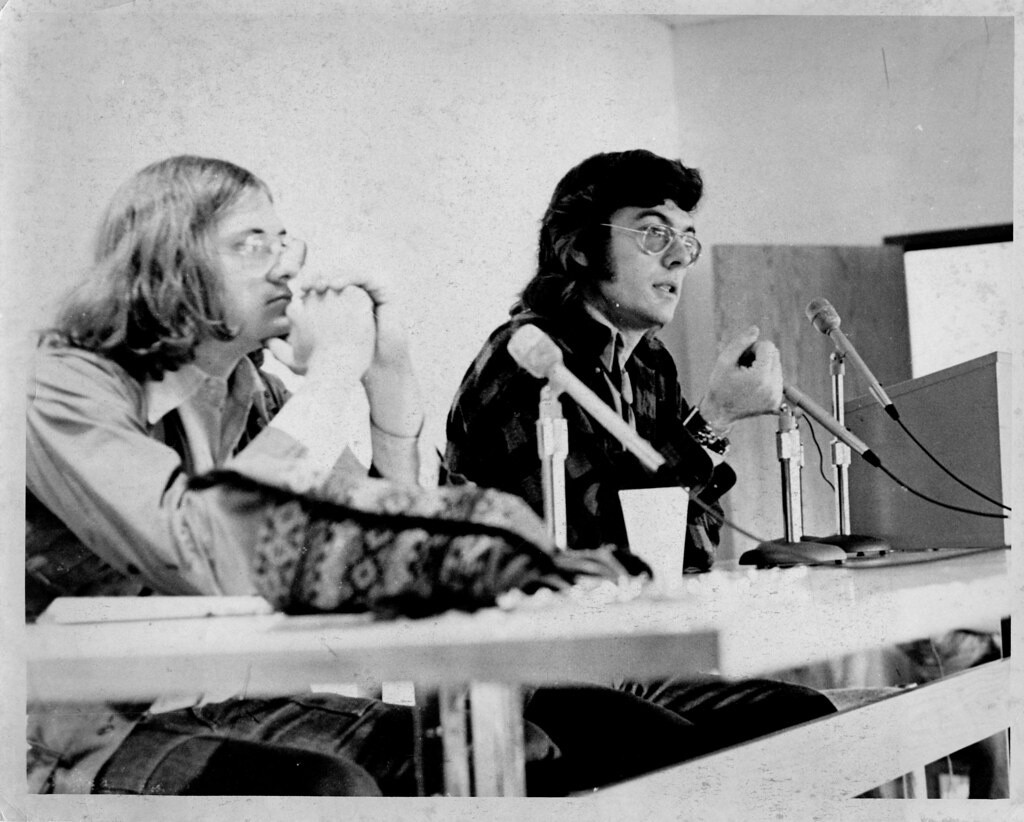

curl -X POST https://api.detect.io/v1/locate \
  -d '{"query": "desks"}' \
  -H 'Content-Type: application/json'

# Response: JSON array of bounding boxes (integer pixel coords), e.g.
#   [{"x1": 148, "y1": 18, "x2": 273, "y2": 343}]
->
[{"x1": 26, "y1": 541, "x2": 1024, "y2": 797}]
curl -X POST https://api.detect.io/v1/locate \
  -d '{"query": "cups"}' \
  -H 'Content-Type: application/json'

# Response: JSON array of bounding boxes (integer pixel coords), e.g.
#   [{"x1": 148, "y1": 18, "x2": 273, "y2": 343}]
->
[{"x1": 617, "y1": 486, "x2": 688, "y2": 583}]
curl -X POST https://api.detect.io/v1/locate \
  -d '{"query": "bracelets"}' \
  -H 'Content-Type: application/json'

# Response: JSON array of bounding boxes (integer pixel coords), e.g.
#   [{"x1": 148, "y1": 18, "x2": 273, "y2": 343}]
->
[{"x1": 682, "y1": 407, "x2": 727, "y2": 455}]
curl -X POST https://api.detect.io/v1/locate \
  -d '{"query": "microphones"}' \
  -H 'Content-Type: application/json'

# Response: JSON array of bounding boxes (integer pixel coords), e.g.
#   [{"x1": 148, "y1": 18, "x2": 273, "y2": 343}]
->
[
  {"x1": 804, "y1": 296, "x2": 900, "y2": 421},
  {"x1": 737, "y1": 348, "x2": 881, "y2": 469},
  {"x1": 507, "y1": 322, "x2": 682, "y2": 485}
]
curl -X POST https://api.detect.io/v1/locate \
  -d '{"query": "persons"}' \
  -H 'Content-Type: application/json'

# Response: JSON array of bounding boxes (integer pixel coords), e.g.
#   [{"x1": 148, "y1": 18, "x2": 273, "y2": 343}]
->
[
  {"x1": 25, "y1": 156, "x2": 563, "y2": 798},
  {"x1": 429, "y1": 147, "x2": 839, "y2": 801}
]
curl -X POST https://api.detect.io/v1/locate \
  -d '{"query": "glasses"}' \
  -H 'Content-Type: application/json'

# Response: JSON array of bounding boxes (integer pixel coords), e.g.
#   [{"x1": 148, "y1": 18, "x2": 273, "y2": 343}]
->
[
  {"x1": 601, "y1": 223, "x2": 703, "y2": 269},
  {"x1": 204, "y1": 235, "x2": 306, "y2": 275}
]
